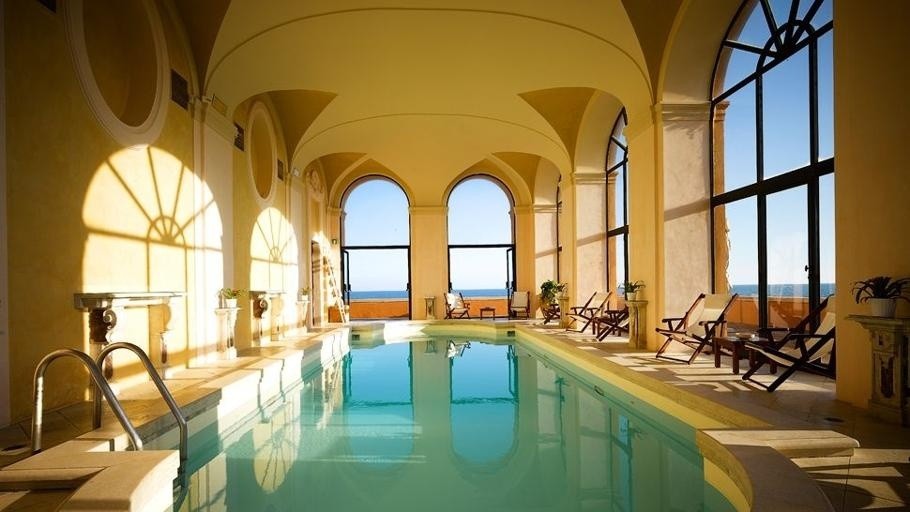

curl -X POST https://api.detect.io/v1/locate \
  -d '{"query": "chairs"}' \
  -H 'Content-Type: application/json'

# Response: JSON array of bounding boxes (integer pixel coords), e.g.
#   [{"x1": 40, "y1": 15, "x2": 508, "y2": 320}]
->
[
  {"x1": 566, "y1": 292, "x2": 638, "y2": 341},
  {"x1": 740, "y1": 293, "x2": 836, "y2": 392},
  {"x1": 543, "y1": 304, "x2": 560, "y2": 324},
  {"x1": 508, "y1": 291, "x2": 530, "y2": 320},
  {"x1": 444, "y1": 292, "x2": 470, "y2": 319},
  {"x1": 656, "y1": 293, "x2": 739, "y2": 365}
]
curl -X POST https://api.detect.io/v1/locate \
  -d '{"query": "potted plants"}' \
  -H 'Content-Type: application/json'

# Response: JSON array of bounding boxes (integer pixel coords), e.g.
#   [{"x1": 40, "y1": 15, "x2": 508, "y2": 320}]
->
[
  {"x1": 541, "y1": 280, "x2": 565, "y2": 302},
  {"x1": 618, "y1": 280, "x2": 646, "y2": 300},
  {"x1": 851, "y1": 276, "x2": 910, "y2": 317},
  {"x1": 220, "y1": 288, "x2": 242, "y2": 307},
  {"x1": 299, "y1": 286, "x2": 310, "y2": 300}
]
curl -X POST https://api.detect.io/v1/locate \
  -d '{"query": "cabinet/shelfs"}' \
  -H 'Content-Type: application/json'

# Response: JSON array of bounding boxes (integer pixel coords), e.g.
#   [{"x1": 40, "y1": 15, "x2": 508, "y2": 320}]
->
[{"x1": 330, "y1": 306, "x2": 350, "y2": 322}]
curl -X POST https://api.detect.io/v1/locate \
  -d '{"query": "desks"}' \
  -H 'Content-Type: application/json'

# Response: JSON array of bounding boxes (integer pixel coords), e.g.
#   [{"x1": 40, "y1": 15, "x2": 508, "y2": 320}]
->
[
  {"x1": 480, "y1": 307, "x2": 495, "y2": 320},
  {"x1": 712, "y1": 336, "x2": 778, "y2": 374}
]
